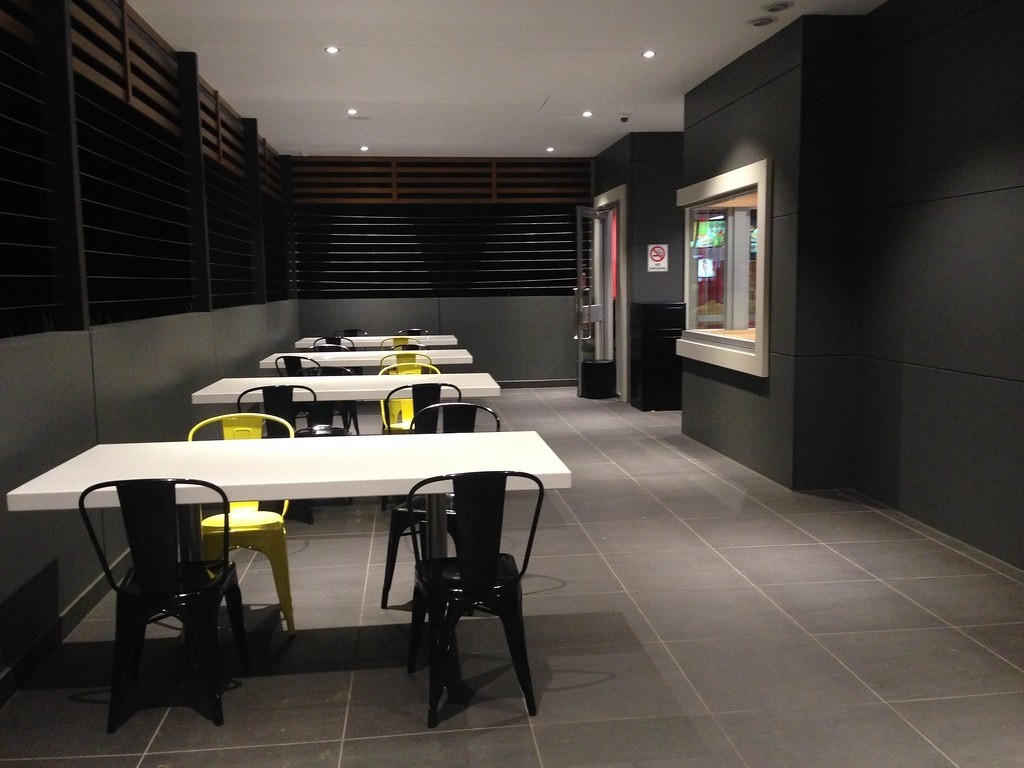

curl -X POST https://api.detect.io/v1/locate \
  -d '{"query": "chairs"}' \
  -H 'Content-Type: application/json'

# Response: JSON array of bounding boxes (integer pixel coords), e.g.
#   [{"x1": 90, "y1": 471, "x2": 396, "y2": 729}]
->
[
  {"x1": 406, "y1": 471, "x2": 544, "y2": 728},
  {"x1": 236, "y1": 356, "x2": 352, "y2": 525},
  {"x1": 333, "y1": 329, "x2": 367, "y2": 336},
  {"x1": 381, "y1": 403, "x2": 501, "y2": 608},
  {"x1": 306, "y1": 336, "x2": 355, "y2": 351},
  {"x1": 187, "y1": 412, "x2": 294, "y2": 636},
  {"x1": 378, "y1": 352, "x2": 461, "y2": 511},
  {"x1": 380, "y1": 337, "x2": 430, "y2": 351},
  {"x1": 396, "y1": 328, "x2": 430, "y2": 335},
  {"x1": 78, "y1": 478, "x2": 246, "y2": 735}
]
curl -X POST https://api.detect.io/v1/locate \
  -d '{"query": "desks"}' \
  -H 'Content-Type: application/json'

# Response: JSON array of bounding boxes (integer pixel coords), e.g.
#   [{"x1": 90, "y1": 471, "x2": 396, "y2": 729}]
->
[
  {"x1": 191, "y1": 372, "x2": 500, "y2": 405},
  {"x1": 6, "y1": 431, "x2": 571, "y2": 702},
  {"x1": 259, "y1": 349, "x2": 473, "y2": 369},
  {"x1": 295, "y1": 335, "x2": 458, "y2": 349}
]
